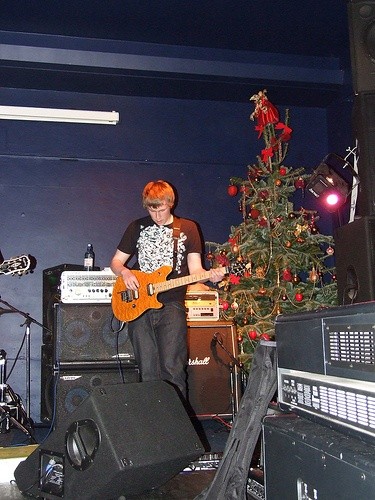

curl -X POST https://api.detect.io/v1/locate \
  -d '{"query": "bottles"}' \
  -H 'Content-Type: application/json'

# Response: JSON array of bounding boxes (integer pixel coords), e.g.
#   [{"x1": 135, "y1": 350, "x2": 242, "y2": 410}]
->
[{"x1": 84, "y1": 243, "x2": 95, "y2": 271}]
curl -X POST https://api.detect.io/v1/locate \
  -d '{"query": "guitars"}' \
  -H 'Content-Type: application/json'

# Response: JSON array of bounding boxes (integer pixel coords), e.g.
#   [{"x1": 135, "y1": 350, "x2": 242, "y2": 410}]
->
[
  {"x1": 111, "y1": 260, "x2": 254, "y2": 323},
  {"x1": 0, "y1": 254, "x2": 37, "y2": 276}
]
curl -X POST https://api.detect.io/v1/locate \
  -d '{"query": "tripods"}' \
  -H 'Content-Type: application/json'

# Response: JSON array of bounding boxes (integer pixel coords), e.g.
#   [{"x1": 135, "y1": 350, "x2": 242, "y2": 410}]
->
[{"x1": 0, "y1": 349, "x2": 32, "y2": 440}]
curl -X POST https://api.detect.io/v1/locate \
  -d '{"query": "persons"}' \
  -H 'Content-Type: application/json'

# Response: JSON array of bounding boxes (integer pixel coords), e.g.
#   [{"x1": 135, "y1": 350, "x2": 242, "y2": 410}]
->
[{"x1": 111, "y1": 180, "x2": 224, "y2": 400}]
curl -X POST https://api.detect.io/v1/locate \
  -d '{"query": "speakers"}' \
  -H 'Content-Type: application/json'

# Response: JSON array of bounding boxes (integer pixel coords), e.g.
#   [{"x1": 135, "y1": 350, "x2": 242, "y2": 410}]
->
[
  {"x1": 335, "y1": 216, "x2": 375, "y2": 306},
  {"x1": 260, "y1": 412, "x2": 375, "y2": 500},
  {"x1": 43, "y1": 303, "x2": 139, "y2": 362},
  {"x1": 40, "y1": 366, "x2": 140, "y2": 429},
  {"x1": 185, "y1": 321, "x2": 245, "y2": 422},
  {"x1": 13, "y1": 381, "x2": 205, "y2": 500},
  {"x1": 347, "y1": 0, "x2": 375, "y2": 95}
]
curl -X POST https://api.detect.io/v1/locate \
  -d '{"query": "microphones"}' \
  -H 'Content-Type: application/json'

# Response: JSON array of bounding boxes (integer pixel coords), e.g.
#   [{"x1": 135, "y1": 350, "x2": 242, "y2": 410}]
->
[{"x1": 214, "y1": 331, "x2": 218, "y2": 341}]
